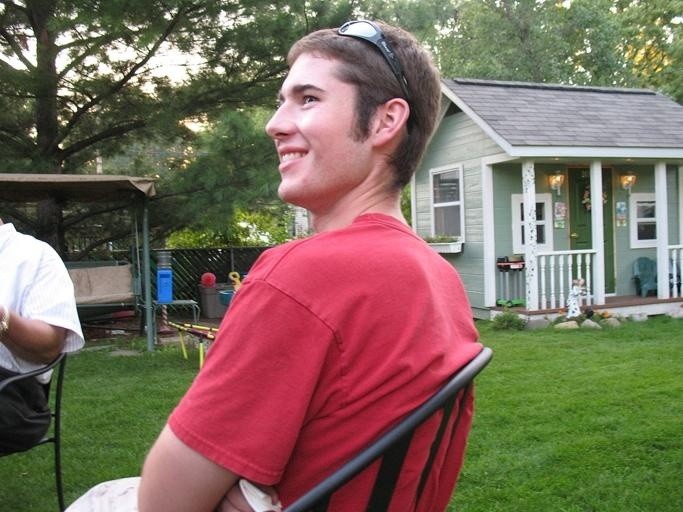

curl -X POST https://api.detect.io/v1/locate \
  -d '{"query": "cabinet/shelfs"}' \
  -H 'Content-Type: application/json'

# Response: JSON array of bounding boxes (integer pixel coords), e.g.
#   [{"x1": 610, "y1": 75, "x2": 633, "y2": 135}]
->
[{"x1": 495, "y1": 261, "x2": 524, "y2": 307}]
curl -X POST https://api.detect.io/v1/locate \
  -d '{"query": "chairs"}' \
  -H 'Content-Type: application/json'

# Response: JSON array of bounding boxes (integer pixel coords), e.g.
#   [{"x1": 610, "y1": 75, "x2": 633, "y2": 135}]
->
[
  {"x1": 0, "y1": 348, "x2": 73, "y2": 512},
  {"x1": 632, "y1": 255, "x2": 671, "y2": 297},
  {"x1": 284, "y1": 347, "x2": 494, "y2": 512}
]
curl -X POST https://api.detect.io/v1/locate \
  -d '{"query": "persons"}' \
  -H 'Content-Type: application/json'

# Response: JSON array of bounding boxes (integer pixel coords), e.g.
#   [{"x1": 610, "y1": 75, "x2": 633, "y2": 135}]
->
[
  {"x1": 0, "y1": 215, "x2": 85, "y2": 458},
  {"x1": 62, "y1": 21, "x2": 479, "y2": 511}
]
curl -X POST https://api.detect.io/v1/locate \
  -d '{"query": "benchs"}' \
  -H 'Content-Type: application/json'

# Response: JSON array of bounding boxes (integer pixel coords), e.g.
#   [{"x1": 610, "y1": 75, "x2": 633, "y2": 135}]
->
[
  {"x1": 60, "y1": 259, "x2": 139, "y2": 310},
  {"x1": 166, "y1": 319, "x2": 217, "y2": 370}
]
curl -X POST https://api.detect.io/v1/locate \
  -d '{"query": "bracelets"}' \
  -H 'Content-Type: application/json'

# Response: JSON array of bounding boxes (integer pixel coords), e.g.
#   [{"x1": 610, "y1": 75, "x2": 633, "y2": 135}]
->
[{"x1": 0, "y1": 303, "x2": 10, "y2": 344}]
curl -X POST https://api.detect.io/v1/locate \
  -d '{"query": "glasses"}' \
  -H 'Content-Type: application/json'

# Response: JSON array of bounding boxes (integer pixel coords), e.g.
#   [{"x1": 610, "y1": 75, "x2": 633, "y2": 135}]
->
[{"x1": 336, "y1": 20, "x2": 416, "y2": 133}]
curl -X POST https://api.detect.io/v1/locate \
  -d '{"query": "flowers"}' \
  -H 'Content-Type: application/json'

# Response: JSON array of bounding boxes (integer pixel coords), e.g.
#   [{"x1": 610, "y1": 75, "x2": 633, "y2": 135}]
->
[{"x1": 581, "y1": 178, "x2": 608, "y2": 211}]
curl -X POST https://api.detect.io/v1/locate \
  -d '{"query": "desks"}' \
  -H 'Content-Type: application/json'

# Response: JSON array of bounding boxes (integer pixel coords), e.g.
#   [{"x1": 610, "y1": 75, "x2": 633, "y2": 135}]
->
[{"x1": 153, "y1": 299, "x2": 201, "y2": 326}]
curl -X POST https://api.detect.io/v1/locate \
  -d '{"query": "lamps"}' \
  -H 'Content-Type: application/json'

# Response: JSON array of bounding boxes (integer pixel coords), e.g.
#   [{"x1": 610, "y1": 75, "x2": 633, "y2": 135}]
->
[
  {"x1": 621, "y1": 170, "x2": 636, "y2": 190},
  {"x1": 548, "y1": 168, "x2": 564, "y2": 191}
]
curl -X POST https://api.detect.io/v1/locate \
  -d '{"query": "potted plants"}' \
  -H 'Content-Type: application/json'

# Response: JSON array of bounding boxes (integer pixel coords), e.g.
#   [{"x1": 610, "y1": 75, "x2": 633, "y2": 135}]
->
[{"x1": 421, "y1": 232, "x2": 463, "y2": 253}]
